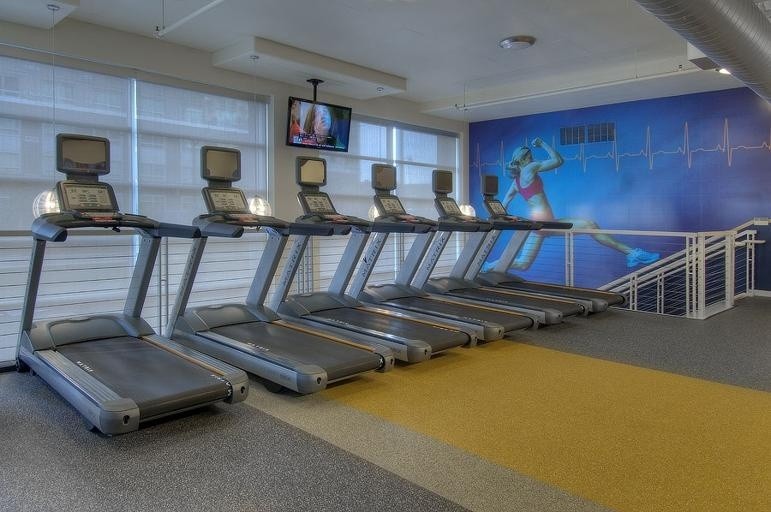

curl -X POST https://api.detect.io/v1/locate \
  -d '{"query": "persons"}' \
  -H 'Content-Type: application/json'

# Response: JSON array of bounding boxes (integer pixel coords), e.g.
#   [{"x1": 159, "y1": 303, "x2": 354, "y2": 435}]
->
[
  {"x1": 290, "y1": 99, "x2": 307, "y2": 145},
  {"x1": 303, "y1": 103, "x2": 346, "y2": 149},
  {"x1": 472, "y1": 136, "x2": 664, "y2": 277}
]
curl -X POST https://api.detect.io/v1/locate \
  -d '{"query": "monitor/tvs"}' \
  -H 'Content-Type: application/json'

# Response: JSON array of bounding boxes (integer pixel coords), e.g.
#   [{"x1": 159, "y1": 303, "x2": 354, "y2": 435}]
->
[
  {"x1": 432, "y1": 169, "x2": 452, "y2": 199},
  {"x1": 481, "y1": 174, "x2": 498, "y2": 200},
  {"x1": 286, "y1": 96, "x2": 352, "y2": 153},
  {"x1": 371, "y1": 163, "x2": 397, "y2": 196},
  {"x1": 200, "y1": 146, "x2": 241, "y2": 188},
  {"x1": 56, "y1": 133, "x2": 110, "y2": 182},
  {"x1": 294, "y1": 156, "x2": 327, "y2": 192}
]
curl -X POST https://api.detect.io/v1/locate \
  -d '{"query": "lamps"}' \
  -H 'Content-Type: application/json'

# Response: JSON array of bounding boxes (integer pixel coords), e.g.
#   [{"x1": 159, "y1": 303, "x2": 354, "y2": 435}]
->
[
  {"x1": 500, "y1": 34, "x2": 537, "y2": 54},
  {"x1": 459, "y1": 83, "x2": 477, "y2": 217},
  {"x1": 33, "y1": 5, "x2": 61, "y2": 219},
  {"x1": 368, "y1": 87, "x2": 385, "y2": 222},
  {"x1": 244, "y1": 57, "x2": 272, "y2": 229}
]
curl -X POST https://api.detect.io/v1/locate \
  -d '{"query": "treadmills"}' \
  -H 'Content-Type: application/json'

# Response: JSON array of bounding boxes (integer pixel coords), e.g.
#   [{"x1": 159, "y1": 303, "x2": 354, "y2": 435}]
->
[
  {"x1": 15, "y1": 131, "x2": 251, "y2": 437},
  {"x1": 465, "y1": 175, "x2": 628, "y2": 315},
  {"x1": 411, "y1": 169, "x2": 592, "y2": 326},
  {"x1": 264, "y1": 156, "x2": 479, "y2": 365},
  {"x1": 161, "y1": 145, "x2": 395, "y2": 394},
  {"x1": 349, "y1": 164, "x2": 541, "y2": 344}
]
